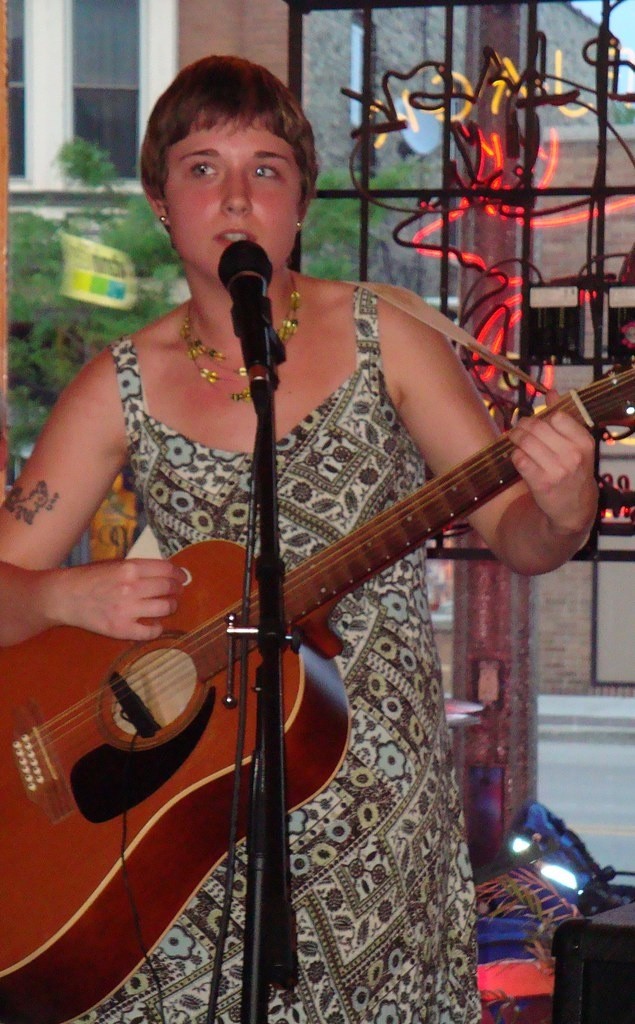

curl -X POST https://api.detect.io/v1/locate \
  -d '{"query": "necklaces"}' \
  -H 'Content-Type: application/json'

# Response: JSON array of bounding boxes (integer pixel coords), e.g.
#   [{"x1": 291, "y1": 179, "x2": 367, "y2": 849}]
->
[{"x1": 181, "y1": 272, "x2": 299, "y2": 400}]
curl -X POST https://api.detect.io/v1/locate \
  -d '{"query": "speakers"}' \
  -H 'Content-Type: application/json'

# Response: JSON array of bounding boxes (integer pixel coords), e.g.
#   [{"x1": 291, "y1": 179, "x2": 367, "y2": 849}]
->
[{"x1": 552, "y1": 902, "x2": 635, "y2": 1024}]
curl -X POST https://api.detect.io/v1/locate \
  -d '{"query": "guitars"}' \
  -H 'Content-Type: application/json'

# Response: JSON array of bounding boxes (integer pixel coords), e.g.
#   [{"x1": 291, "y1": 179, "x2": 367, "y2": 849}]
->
[{"x1": 0, "y1": 348, "x2": 635, "y2": 1024}]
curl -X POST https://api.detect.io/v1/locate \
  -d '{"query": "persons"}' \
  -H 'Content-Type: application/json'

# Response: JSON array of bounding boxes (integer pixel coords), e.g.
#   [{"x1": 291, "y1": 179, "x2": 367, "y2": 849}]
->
[{"x1": 1, "y1": 56, "x2": 599, "y2": 1024}]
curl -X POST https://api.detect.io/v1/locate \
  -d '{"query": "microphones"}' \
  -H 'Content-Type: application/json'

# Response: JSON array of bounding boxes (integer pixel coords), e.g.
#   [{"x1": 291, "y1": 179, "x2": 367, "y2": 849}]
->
[{"x1": 217, "y1": 238, "x2": 272, "y2": 416}]
